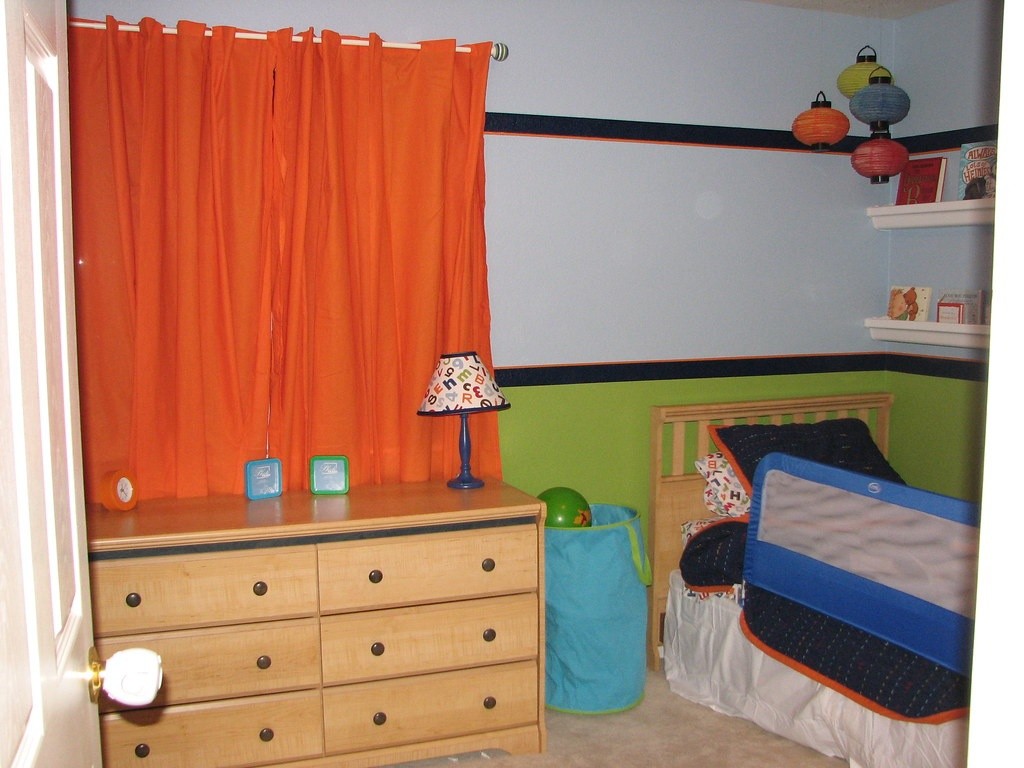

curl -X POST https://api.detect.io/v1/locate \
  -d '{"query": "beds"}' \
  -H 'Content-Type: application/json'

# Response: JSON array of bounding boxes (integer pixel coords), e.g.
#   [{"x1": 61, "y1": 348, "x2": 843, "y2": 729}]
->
[{"x1": 647, "y1": 391, "x2": 981, "y2": 768}]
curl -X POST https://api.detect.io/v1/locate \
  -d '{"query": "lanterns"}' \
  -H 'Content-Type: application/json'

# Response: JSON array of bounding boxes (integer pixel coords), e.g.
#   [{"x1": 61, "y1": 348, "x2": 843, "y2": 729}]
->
[
  {"x1": 837, "y1": 46, "x2": 911, "y2": 184},
  {"x1": 792, "y1": 93, "x2": 849, "y2": 153}
]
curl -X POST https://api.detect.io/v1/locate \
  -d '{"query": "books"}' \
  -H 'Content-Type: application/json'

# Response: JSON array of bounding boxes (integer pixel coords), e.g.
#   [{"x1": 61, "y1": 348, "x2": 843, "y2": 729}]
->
[
  {"x1": 897, "y1": 157, "x2": 946, "y2": 204},
  {"x1": 958, "y1": 142, "x2": 996, "y2": 199}
]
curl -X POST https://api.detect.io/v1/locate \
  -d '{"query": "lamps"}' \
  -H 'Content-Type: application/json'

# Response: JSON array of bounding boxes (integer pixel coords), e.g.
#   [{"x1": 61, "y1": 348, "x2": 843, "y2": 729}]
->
[{"x1": 417, "y1": 352, "x2": 511, "y2": 490}]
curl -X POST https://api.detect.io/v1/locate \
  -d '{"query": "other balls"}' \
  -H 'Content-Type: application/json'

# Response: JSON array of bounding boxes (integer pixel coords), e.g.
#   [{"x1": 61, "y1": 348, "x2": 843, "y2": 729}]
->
[{"x1": 537, "y1": 486, "x2": 592, "y2": 530}]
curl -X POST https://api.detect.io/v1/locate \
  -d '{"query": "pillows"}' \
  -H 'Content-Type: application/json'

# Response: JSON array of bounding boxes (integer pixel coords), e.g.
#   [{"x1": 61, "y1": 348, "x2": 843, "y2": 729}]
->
[
  {"x1": 694, "y1": 452, "x2": 750, "y2": 517},
  {"x1": 707, "y1": 418, "x2": 907, "y2": 499}
]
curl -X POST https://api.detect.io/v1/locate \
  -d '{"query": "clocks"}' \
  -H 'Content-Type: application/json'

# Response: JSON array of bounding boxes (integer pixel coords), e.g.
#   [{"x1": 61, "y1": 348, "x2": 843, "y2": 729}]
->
[{"x1": 99, "y1": 469, "x2": 139, "y2": 512}]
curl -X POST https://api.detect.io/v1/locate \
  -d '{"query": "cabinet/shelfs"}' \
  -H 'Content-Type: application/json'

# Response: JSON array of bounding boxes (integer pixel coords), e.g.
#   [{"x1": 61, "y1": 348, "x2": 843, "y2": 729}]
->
[
  {"x1": 87, "y1": 475, "x2": 548, "y2": 768},
  {"x1": 863, "y1": 199, "x2": 995, "y2": 350}
]
